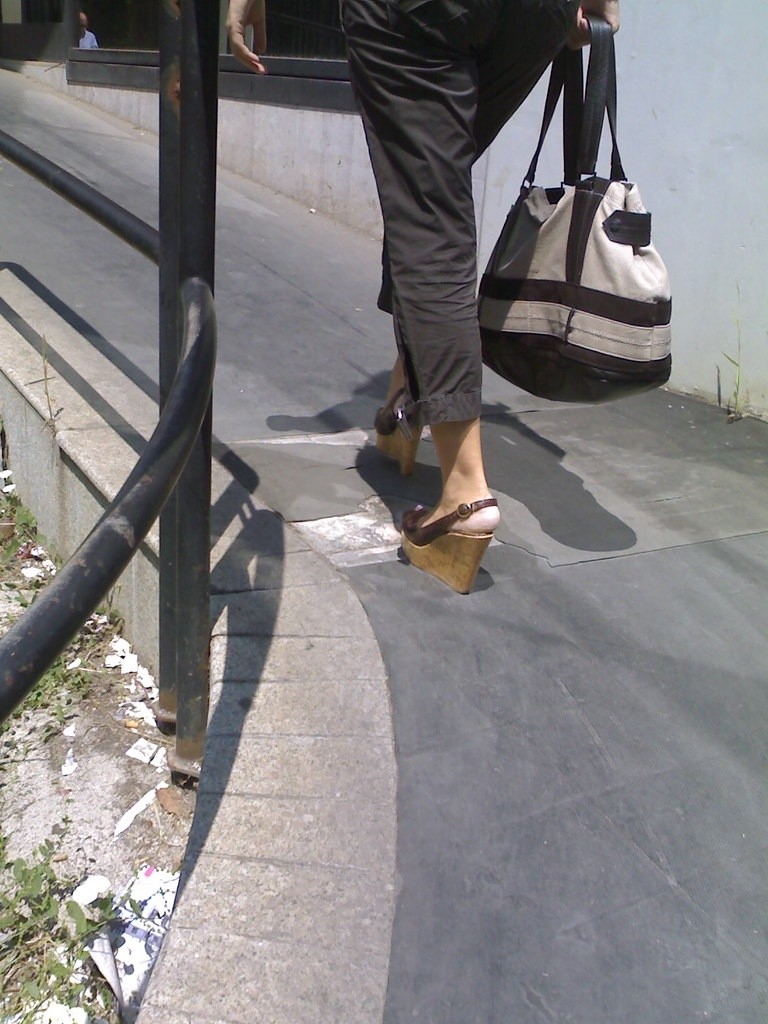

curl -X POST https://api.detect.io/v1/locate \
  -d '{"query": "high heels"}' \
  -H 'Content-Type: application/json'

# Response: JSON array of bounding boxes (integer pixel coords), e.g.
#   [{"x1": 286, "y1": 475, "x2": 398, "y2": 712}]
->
[
  {"x1": 400, "y1": 498, "x2": 499, "y2": 593},
  {"x1": 373, "y1": 387, "x2": 424, "y2": 478}
]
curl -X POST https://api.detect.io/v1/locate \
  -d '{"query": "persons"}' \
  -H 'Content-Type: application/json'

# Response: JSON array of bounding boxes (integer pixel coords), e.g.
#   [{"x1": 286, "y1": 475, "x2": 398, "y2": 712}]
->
[
  {"x1": 225, "y1": 0, "x2": 619, "y2": 596},
  {"x1": 80, "y1": 13, "x2": 98, "y2": 48}
]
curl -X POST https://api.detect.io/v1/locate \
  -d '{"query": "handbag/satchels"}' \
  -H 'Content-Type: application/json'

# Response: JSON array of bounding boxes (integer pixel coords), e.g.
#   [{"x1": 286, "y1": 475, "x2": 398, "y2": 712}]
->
[{"x1": 478, "y1": 11, "x2": 674, "y2": 404}]
